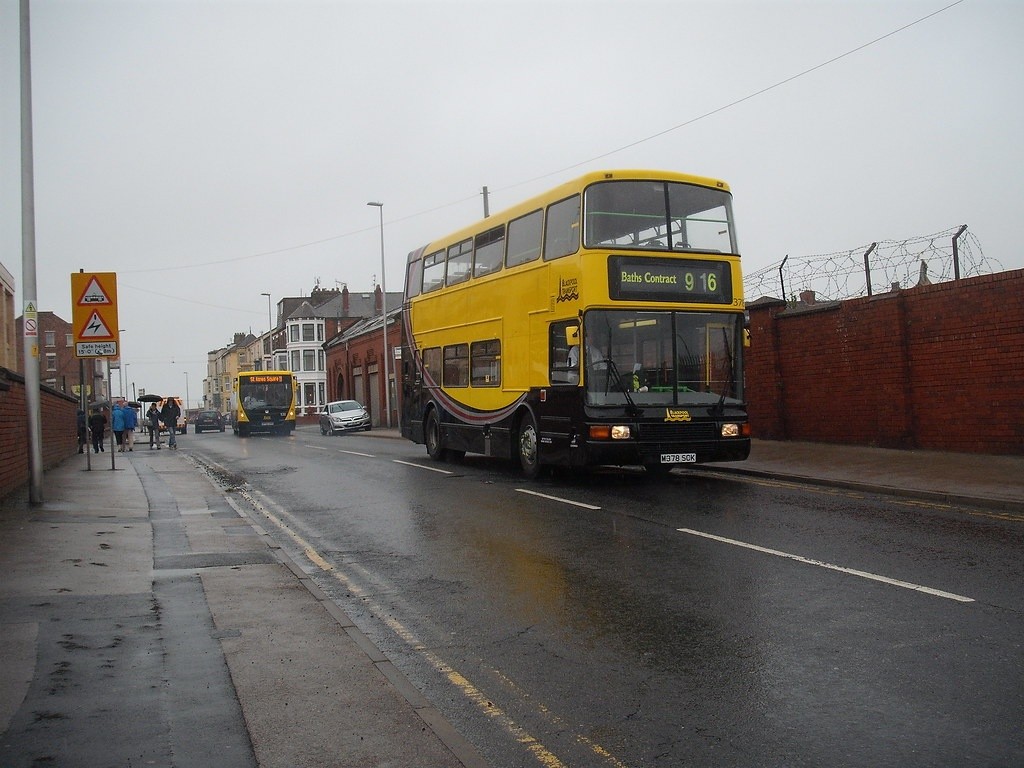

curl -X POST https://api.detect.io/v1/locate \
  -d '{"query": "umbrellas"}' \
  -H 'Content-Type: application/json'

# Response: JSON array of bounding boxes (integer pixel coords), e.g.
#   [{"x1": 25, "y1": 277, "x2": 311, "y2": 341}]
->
[
  {"x1": 126, "y1": 401, "x2": 143, "y2": 408},
  {"x1": 88, "y1": 400, "x2": 110, "y2": 408},
  {"x1": 137, "y1": 394, "x2": 163, "y2": 407}
]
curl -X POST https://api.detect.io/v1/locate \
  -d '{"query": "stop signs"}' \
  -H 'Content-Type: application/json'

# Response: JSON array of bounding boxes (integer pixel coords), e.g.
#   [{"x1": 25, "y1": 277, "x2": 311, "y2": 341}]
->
[{"x1": 25, "y1": 319, "x2": 36, "y2": 331}]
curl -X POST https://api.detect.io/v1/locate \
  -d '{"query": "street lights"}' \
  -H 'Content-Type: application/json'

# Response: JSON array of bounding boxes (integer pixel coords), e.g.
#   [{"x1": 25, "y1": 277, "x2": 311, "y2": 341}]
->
[
  {"x1": 124, "y1": 362, "x2": 131, "y2": 401},
  {"x1": 183, "y1": 371, "x2": 190, "y2": 425},
  {"x1": 261, "y1": 292, "x2": 274, "y2": 370},
  {"x1": 366, "y1": 200, "x2": 391, "y2": 429}
]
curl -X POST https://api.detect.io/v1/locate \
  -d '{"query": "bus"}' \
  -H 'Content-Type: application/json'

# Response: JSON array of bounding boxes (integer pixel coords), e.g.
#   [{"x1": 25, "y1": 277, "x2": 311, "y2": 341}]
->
[
  {"x1": 399, "y1": 168, "x2": 753, "y2": 482},
  {"x1": 230, "y1": 370, "x2": 297, "y2": 437}
]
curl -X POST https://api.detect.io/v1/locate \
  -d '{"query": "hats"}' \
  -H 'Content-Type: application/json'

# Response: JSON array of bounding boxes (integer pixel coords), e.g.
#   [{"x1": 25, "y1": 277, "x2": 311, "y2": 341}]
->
[{"x1": 123, "y1": 402, "x2": 128, "y2": 406}]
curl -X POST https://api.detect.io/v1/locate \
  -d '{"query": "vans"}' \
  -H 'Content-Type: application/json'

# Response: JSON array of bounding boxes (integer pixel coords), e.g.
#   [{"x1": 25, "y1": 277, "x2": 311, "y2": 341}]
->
[{"x1": 157, "y1": 396, "x2": 187, "y2": 435}]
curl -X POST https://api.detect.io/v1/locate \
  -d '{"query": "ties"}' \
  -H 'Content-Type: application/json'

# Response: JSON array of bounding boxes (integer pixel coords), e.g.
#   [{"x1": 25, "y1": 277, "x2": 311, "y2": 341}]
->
[
  {"x1": 250, "y1": 398, "x2": 251, "y2": 404},
  {"x1": 588, "y1": 347, "x2": 593, "y2": 372}
]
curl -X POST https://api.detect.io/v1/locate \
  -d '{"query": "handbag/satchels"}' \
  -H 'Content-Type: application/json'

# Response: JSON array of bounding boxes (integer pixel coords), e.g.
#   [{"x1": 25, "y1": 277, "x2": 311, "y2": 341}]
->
[{"x1": 147, "y1": 421, "x2": 153, "y2": 427}]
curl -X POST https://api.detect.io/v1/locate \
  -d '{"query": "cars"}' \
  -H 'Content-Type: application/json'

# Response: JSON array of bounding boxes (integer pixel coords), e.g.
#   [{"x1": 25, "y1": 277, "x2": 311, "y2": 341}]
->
[
  {"x1": 193, "y1": 409, "x2": 227, "y2": 433},
  {"x1": 318, "y1": 400, "x2": 372, "y2": 436}
]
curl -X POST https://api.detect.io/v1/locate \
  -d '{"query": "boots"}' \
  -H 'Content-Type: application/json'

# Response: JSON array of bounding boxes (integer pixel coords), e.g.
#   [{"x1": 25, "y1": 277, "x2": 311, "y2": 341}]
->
[{"x1": 118, "y1": 445, "x2": 123, "y2": 452}]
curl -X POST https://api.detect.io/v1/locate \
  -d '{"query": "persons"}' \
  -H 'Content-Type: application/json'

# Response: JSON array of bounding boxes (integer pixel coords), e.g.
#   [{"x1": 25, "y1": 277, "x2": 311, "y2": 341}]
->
[
  {"x1": 161, "y1": 397, "x2": 181, "y2": 450},
  {"x1": 566, "y1": 326, "x2": 611, "y2": 385},
  {"x1": 113, "y1": 402, "x2": 137, "y2": 451},
  {"x1": 88, "y1": 408, "x2": 108, "y2": 453},
  {"x1": 77, "y1": 409, "x2": 90, "y2": 453},
  {"x1": 146, "y1": 403, "x2": 163, "y2": 449},
  {"x1": 244, "y1": 390, "x2": 258, "y2": 406}
]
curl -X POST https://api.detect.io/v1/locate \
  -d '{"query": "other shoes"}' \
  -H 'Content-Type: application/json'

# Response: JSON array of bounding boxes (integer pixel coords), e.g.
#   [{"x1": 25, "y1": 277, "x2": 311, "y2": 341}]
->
[
  {"x1": 79, "y1": 452, "x2": 84, "y2": 454},
  {"x1": 100, "y1": 446, "x2": 104, "y2": 452},
  {"x1": 157, "y1": 447, "x2": 161, "y2": 449},
  {"x1": 95, "y1": 451, "x2": 98, "y2": 453},
  {"x1": 129, "y1": 449, "x2": 132, "y2": 451},
  {"x1": 170, "y1": 443, "x2": 177, "y2": 450}
]
